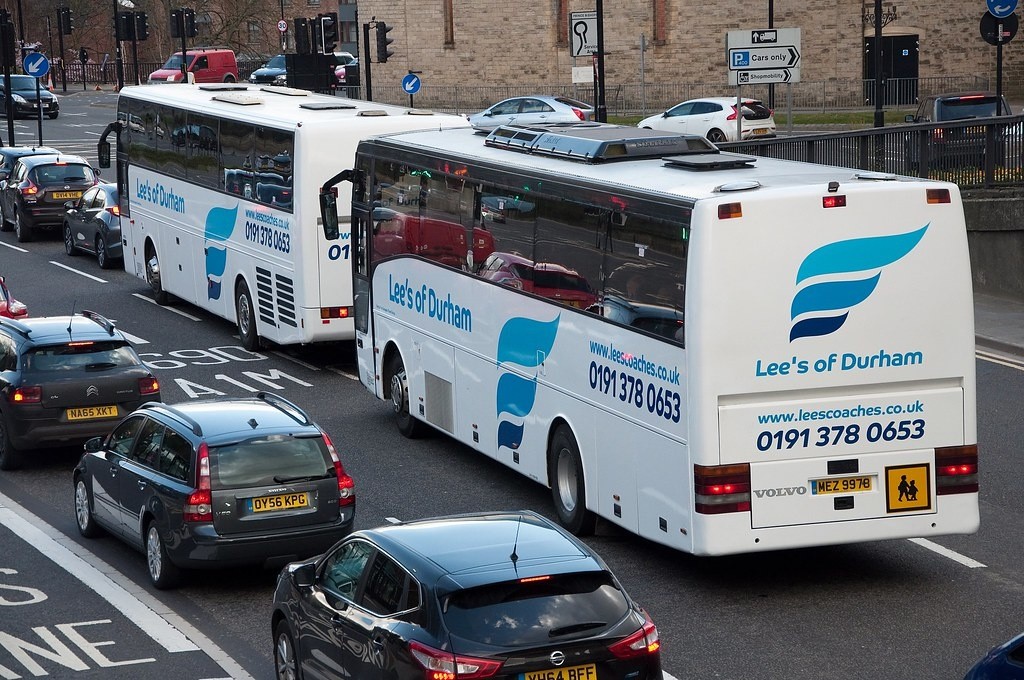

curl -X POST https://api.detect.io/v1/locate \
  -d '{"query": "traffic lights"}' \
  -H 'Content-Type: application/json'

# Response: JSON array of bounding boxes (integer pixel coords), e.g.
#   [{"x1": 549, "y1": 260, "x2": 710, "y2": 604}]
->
[
  {"x1": 62, "y1": 7, "x2": 76, "y2": 35},
  {"x1": 376, "y1": 21, "x2": 395, "y2": 64},
  {"x1": 321, "y1": 17, "x2": 337, "y2": 55},
  {"x1": 0, "y1": 8, "x2": 12, "y2": 24}
]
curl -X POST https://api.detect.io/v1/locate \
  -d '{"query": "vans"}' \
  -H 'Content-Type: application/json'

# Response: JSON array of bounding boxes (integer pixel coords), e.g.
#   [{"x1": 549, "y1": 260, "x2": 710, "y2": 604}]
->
[{"x1": 147, "y1": 44, "x2": 239, "y2": 86}]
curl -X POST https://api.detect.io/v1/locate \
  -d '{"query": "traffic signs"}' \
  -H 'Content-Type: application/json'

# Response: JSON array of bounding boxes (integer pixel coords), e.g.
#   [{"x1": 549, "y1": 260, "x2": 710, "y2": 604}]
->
[{"x1": 726, "y1": 26, "x2": 802, "y2": 87}]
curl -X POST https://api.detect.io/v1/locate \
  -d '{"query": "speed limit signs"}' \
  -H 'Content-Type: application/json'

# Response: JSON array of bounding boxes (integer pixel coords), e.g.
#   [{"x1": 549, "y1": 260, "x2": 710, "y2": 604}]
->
[{"x1": 277, "y1": 19, "x2": 289, "y2": 33}]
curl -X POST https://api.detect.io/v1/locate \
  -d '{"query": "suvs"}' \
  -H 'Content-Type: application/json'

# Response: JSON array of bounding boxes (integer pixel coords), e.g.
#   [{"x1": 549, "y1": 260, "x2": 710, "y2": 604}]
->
[
  {"x1": 902, "y1": 89, "x2": 1015, "y2": 173},
  {"x1": 71, "y1": 388, "x2": 358, "y2": 592},
  {"x1": 0, "y1": 153, "x2": 101, "y2": 244},
  {"x1": 0, "y1": 308, "x2": 164, "y2": 472},
  {"x1": 268, "y1": 508, "x2": 677, "y2": 680}
]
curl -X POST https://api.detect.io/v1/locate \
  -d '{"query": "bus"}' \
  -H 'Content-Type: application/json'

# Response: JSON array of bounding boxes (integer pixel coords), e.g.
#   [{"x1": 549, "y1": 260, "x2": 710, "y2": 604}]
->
[
  {"x1": 316, "y1": 114, "x2": 983, "y2": 566},
  {"x1": 98, "y1": 68, "x2": 474, "y2": 357}
]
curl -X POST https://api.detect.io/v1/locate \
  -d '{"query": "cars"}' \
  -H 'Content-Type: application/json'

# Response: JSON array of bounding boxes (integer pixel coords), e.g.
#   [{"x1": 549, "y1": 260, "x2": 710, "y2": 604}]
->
[
  {"x1": 0, "y1": 73, "x2": 60, "y2": 121},
  {"x1": 61, "y1": 182, "x2": 124, "y2": 270},
  {"x1": 249, "y1": 50, "x2": 360, "y2": 89},
  {"x1": 635, "y1": 95, "x2": 778, "y2": 155},
  {"x1": 465, "y1": 92, "x2": 599, "y2": 136},
  {"x1": 371, "y1": 192, "x2": 685, "y2": 347},
  {"x1": 221, "y1": 153, "x2": 293, "y2": 213},
  {"x1": 0, "y1": 144, "x2": 65, "y2": 233}
]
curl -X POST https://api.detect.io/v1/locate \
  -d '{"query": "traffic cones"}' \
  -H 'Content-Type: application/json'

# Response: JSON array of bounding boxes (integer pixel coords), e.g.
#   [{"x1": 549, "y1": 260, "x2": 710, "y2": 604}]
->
[{"x1": 47, "y1": 73, "x2": 55, "y2": 91}]
